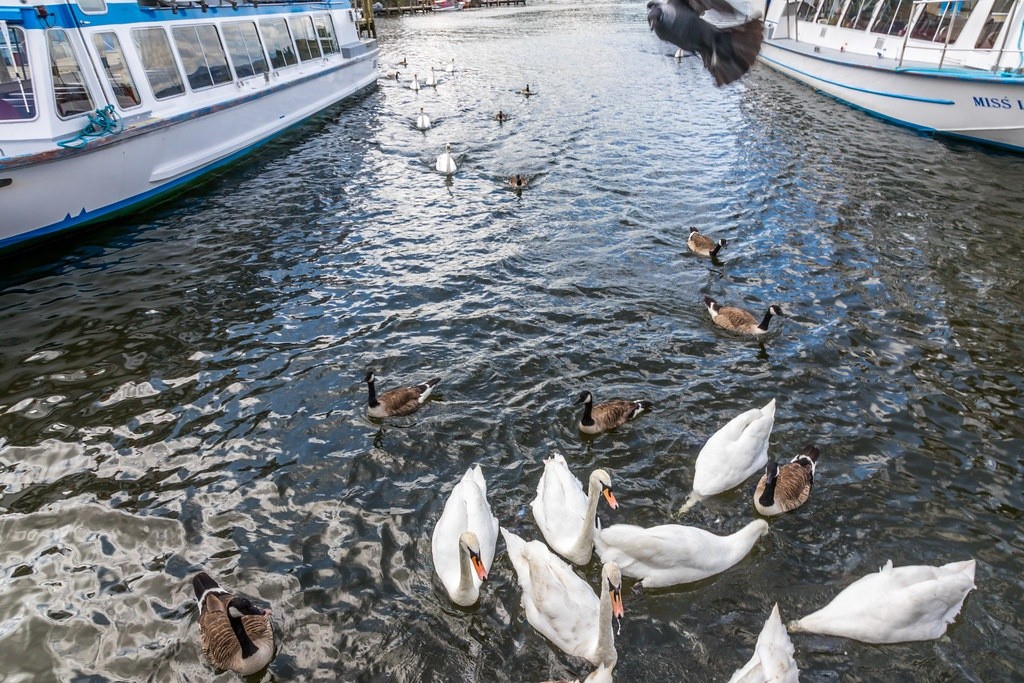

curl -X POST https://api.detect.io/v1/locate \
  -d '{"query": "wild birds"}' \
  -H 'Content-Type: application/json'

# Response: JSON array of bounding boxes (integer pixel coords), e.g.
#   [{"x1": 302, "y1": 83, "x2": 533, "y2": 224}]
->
[{"x1": 642, "y1": 0, "x2": 764, "y2": 88}]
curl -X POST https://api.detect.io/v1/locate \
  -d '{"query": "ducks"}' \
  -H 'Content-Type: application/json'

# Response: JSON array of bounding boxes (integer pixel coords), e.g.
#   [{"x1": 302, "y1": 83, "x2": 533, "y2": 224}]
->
[
  {"x1": 676, "y1": 397, "x2": 776, "y2": 513},
  {"x1": 500, "y1": 525, "x2": 625, "y2": 670},
  {"x1": 495, "y1": 110, "x2": 508, "y2": 121},
  {"x1": 192, "y1": 572, "x2": 274, "y2": 675},
  {"x1": 509, "y1": 174, "x2": 527, "y2": 187},
  {"x1": 432, "y1": 462, "x2": 499, "y2": 607},
  {"x1": 686, "y1": 226, "x2": 727, "y2": 259},
  {"x1": 529, "y1": 452, "x2": 619, "y2": 566},
  {"x1": 357, "y1": 371, "x2": 441, "y2": 417},
  {"x1": 753, "y1": 445, "x2": 820, "y2": 515},
  {"x1": 520, "y1": 84, "x2": 530, "y2": 94},
  {"x1": 435, "y1": 143, "x2": 457, "y2": 173},
  {"x1": 569, "y1": 391, "x2": 654, "y2": 434},
  {"x1": 585, "y1": 516, "x2": 769, "y2": 589},
  {"x1": 788, "y1": 560, "x2": 978, "y2": 645},
  {"x1": 701, "y1": 295, "x2": 787, "y2": 335},
  {"x1": 377, "y1": 57, "x2": 457, "y2": 90},
  {"x1": 416, "y1": 106, "x2": 431, "y2": 130}
]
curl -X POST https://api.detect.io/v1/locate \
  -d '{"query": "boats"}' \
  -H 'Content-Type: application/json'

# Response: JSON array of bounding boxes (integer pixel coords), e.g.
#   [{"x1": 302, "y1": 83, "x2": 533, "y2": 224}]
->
[
  {"x1": 431, "y1": 0, "x2": 466, "y2": 12},
  {"x1": 0, "y1": 0, "x2": 384, "y2": 252},
  {"x1": 755, "y1": 0, "x2": 1024, "y2": 154}
]
